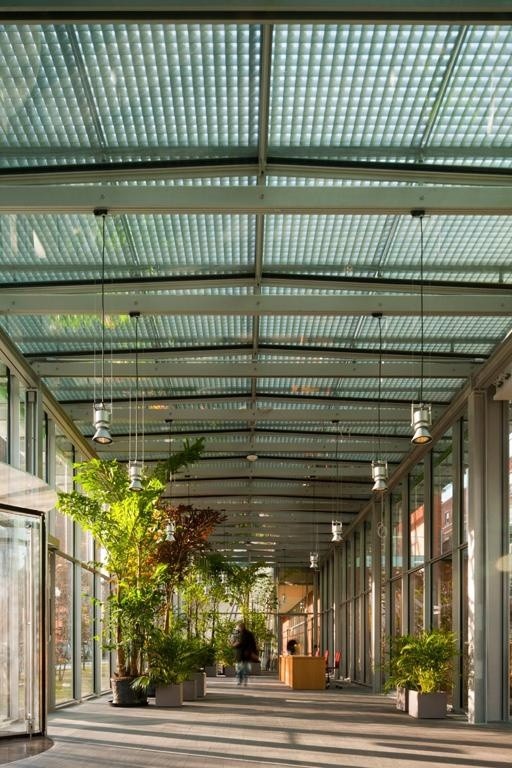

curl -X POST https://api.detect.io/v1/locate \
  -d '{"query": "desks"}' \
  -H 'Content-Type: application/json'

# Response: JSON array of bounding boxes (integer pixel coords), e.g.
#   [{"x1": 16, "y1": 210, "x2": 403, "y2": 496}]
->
[{"x1": 278, "y1": 655, "x2": 325, "y2": 690}]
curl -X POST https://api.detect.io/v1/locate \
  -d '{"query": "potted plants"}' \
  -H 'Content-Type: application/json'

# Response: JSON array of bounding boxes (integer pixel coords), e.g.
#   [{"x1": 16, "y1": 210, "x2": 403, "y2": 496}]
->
[
  {"x1": 368, "y1": 627, "x2": 430, "y2": 711},
  {"x1": 399, "y1": 633, "x2": 468, "y2": 720},
  {"x1": 56, "y1": 435, "x2": 276, "y2": 708}
]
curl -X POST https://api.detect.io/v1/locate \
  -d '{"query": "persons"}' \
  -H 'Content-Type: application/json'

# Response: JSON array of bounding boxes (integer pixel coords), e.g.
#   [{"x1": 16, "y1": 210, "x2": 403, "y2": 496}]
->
[{"x1": 230, "y1": 621, "x2": 259, "y2": 689}]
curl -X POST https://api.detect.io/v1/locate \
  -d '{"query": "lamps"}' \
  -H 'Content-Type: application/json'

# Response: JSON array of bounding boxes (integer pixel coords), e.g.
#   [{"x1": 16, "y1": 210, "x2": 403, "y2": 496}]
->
[
  {"x1": 282, "y1": 548, "x2": 286, "y2": 605},
  {"x1": 309, "y1": 474, "x2": 320, "y2": 569},
  {"x1": 330, "y1": 420, "x2": 345, "y2": 542},
  {"x1": 164, "y1": 418, "x2": 176, "y2": 543},
  {"x1": 408, "y1": 207, "x2": 434, "y2": 445},
  {"x1": 300, "y1": 562, "x2": 305, "y2": 612},
  {"x1": 92, "y1": 208, "x2": 114, "y2": 445},
  {"x1": 370, "y1": 311, "x2": 390, "y2": 491},
  {"x1": 127, "y1": 312, "x2": 145, "y2": 493}
]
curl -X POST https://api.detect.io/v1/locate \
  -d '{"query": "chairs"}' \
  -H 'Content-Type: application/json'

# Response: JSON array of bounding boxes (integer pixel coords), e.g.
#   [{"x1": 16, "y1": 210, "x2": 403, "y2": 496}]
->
[
  {"x1": 315, "y1": 648, "x2": 320, "y2": 656},
  {"x1": 325, "y1": 651, "x2": 342, "y2": 689},
  {"x1": 322, "y1": 651, "x2": 328, "y2": 668}
]
